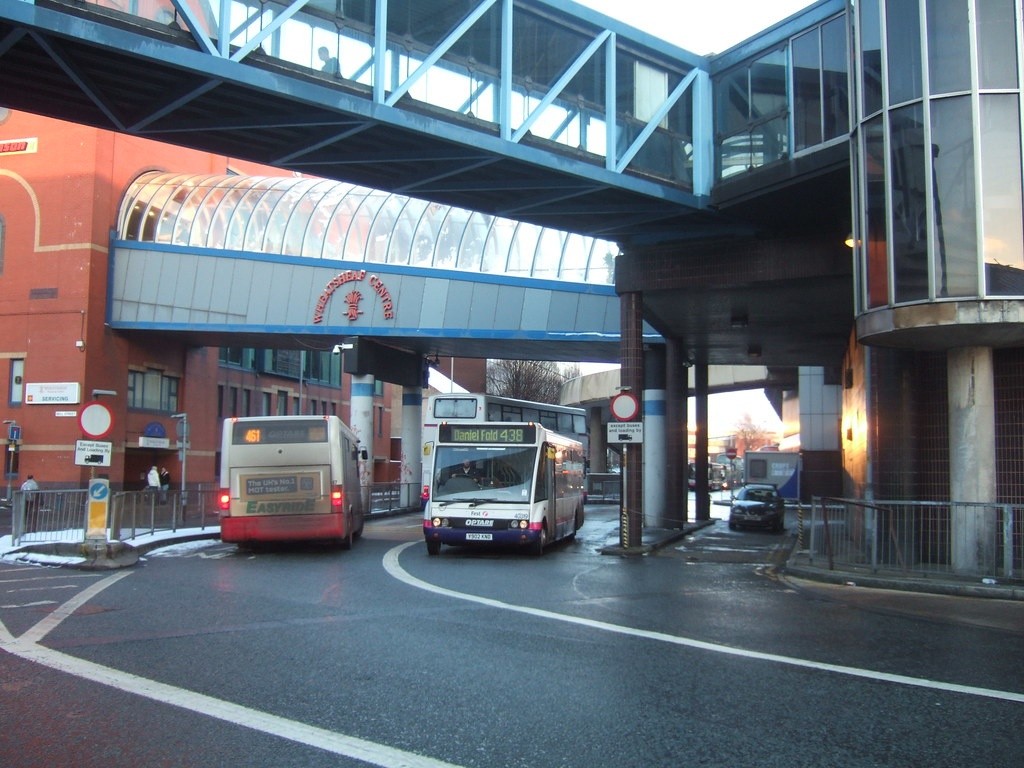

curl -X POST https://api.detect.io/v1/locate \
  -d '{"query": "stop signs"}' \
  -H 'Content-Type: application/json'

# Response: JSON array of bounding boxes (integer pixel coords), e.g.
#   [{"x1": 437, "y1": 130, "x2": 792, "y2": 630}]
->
[{"x1": 726, "y1": 448, "x2": 737, "y2": 459}]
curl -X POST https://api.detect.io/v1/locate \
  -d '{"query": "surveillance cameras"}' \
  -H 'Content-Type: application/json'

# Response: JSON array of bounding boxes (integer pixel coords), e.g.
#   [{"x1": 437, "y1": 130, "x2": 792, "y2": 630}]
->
[{"x1": 333, "y1": 346, "x2": 340, "y2": 355}]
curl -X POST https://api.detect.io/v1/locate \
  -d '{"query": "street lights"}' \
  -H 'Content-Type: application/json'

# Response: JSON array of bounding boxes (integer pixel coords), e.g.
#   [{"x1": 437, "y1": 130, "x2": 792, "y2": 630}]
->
[
  {"x1": 83, "y1": 389, "x2": 118, "y2": 543},
  {"x1": 172, "y1": 414, "x2": 186, "y2": 526},
  {"x1": 3, "y1": 420, "x2": 17, "y2": 505},
  {"x1": 615, "y1": 386, "x2": 632, "y2": 550}
]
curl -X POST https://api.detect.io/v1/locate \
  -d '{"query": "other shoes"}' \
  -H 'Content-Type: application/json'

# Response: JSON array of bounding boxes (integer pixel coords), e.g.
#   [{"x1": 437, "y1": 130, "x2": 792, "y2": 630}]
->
[{"x1": 160, "y1": 501, "x2": 166, "y2": 504}]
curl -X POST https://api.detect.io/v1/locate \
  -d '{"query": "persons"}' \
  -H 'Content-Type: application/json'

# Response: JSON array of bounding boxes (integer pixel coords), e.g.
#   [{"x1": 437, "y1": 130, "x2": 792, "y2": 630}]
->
[
  {"x1": 449, "y1": 458, "x2": 479, "y2": 483},
  {"x1": 20, "y1": 475, "x2": 38, "y2": 527},
  {"x1": 159, "y1": 466, "x2": 169, "y2": 505},
  {"x1": 146, "y1": 466, "x2": 163, "y2": 506}
]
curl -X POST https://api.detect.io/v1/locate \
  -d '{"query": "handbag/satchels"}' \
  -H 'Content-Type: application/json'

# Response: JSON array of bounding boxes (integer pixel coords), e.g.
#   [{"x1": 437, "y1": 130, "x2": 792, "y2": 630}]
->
[{"x1": 35, "y1": 490, "x2": 44, "y2": 506}]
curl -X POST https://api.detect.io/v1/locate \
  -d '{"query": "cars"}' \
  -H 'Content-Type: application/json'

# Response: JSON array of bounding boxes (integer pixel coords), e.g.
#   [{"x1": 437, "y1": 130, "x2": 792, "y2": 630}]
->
[{"x1": 728, "y1": 483, "x2": 786, "y2": 533}]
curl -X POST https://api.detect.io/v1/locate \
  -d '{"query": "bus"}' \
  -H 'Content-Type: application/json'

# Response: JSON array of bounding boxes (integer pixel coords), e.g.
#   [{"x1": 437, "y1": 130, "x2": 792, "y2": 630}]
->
[
  {"x1": 421, "y1": 420, "x2": 585, "y2": 554},
  {"x1": 219, "y1": 415, "x2": 369, "y2": 551},
  {"x1": 419, "y1": 392, "x2": 591, "y2": 509},
  {"x1": 717, "y1": 453, "x2": 744, "y2": 481},
  {"x1": 688, "y1": 461, "x2": 725, "y2": 491}
]
